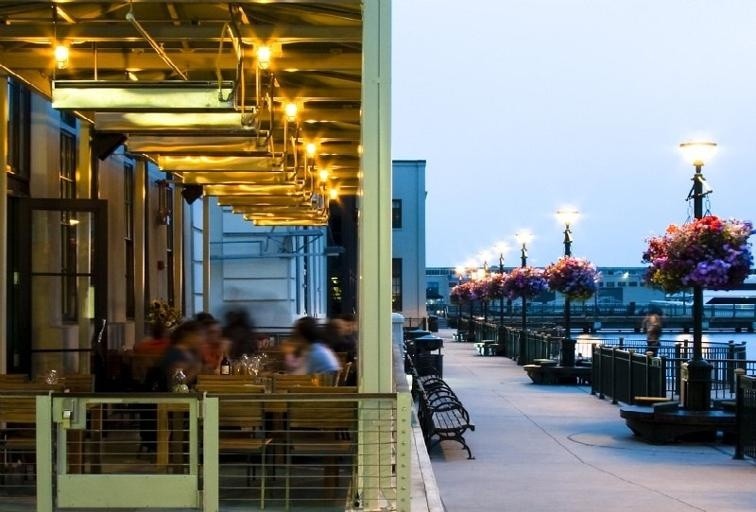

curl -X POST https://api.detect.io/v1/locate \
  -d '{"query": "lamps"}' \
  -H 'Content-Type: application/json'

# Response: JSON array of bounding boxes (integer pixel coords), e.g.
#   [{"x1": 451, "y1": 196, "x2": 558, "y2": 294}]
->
[{"x1": 50, "y1": 53, "x2": 327, "y2": 226}]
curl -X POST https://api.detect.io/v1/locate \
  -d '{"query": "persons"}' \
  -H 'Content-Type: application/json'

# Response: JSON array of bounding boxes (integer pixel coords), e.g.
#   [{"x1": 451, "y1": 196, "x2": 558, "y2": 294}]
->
[{"x1": 143, "y1": 308, "x2": 359, "y2": 397}]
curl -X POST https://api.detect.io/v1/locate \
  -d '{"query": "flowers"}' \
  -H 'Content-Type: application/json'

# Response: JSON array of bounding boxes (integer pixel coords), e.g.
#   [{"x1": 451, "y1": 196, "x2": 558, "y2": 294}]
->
[
  {"x1": 444, "y1": 259, "x2": 598, "y2": 307},
  {"x1": 644, "y1": 216, "x2": 752, "y2": 298},
  {"x1": 146, "y1": 297, "x2": 182, "y2": 327}
]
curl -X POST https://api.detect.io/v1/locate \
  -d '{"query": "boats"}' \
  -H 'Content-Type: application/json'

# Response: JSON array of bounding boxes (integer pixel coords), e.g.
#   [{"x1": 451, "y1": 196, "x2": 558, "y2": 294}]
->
[{"x1": 648, "y1": 265, "x2": 756, "y2": 322}]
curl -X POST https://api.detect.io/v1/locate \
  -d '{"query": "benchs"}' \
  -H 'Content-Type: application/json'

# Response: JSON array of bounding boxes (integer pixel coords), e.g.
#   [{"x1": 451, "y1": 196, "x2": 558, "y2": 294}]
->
[
  {"x1": 523, "y1": 355, "x2": 592, "y2": 384},
  {"x1": 473, "y1": 340, "x2": 501, "y2": 355},
  {"x1": 403, "y1": 356, "x2": 476, "y2": 460},
  {"x1": 620, "y1": 395, "x2": 738, "y2": 446}
]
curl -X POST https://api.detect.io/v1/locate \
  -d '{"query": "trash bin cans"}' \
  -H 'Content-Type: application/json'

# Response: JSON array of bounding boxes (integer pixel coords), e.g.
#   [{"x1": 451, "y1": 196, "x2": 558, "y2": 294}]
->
[
  {"x1": 404, "y1": 326, "x2": 443, "y2": 379},
  {"x1": 678, "y1": 357, "x2": 713, "y2": 411},
  {"x1": 560, "y1": 339, "x2": 576, "y2": 367},
  {"x1": 428, "y1": 317, "x2": 439, "y2": 332}
]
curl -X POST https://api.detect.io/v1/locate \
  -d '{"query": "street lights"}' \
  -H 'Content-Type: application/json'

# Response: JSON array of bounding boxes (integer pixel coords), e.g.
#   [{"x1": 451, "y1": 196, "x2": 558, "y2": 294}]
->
[
  {"x1": 453, "y1": 243, "x2": 510, "y2": 357},
  {"x1": 679, "y1": 139, "x2": 719, "y2": 411},
  {"x1": 512, "y1": 231, "x2": 534, "y2": 365},
  {"x1": 554, "y1": 207, "x2": 581, "y2": 368}
]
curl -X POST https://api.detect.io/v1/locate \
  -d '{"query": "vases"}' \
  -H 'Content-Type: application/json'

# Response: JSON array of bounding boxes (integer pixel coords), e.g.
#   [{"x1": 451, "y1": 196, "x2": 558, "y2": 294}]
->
[{"x1": 151, "y1": 324, "x2": 170, "y2": 340}]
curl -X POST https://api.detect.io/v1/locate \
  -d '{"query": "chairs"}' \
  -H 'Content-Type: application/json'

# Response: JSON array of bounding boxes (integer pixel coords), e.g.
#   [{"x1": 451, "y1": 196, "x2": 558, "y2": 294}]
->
[
  {"x1": 126, "y1": 348, "x2": 358, "y2": 500},
  {"x1": 0, "y1": 372, "x2": 105, "y2": 474}
]
